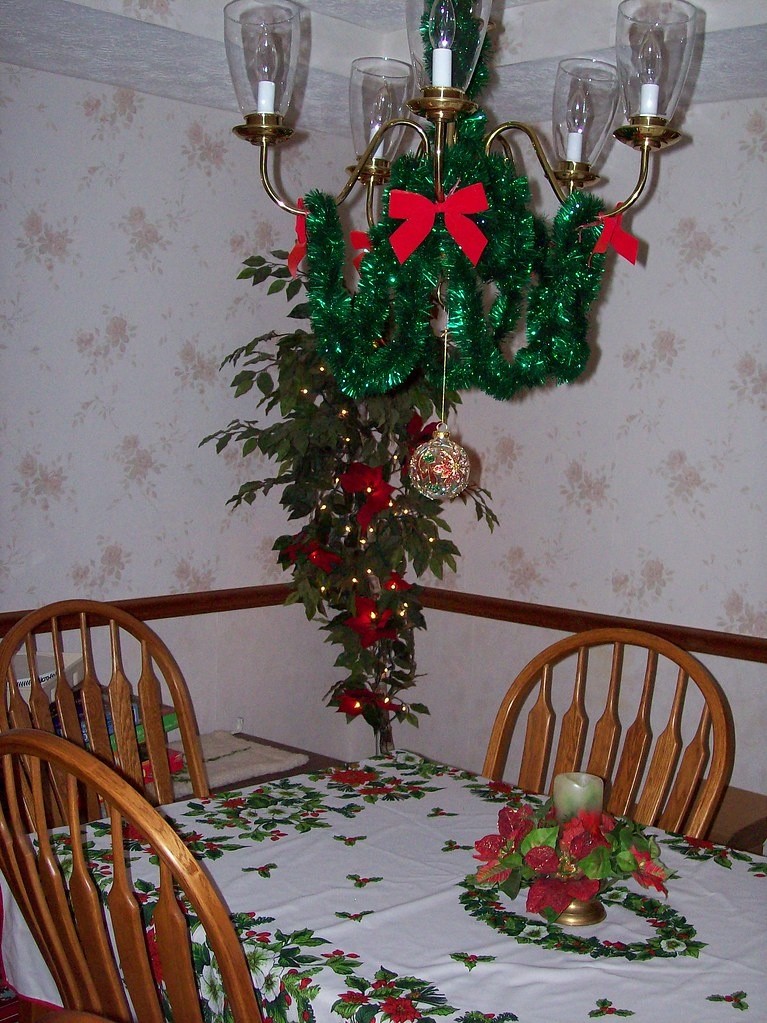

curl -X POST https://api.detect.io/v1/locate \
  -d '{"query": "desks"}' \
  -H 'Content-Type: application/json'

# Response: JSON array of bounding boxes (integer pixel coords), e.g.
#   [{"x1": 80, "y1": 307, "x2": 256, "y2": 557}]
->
[{"x1": 0, "y1": 748, "x2": 767, "y2": 1023}]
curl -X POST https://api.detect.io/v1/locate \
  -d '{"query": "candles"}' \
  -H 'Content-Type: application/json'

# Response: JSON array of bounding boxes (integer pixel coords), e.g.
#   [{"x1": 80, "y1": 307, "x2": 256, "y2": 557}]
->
[{"x1": 552, "y1": 771, "x2": 603, "y2": 828}]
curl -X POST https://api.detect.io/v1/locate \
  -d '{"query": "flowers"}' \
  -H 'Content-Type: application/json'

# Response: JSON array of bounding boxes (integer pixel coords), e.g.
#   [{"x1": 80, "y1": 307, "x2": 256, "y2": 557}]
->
[
  {"x1": 472, "y1": 798, "x2": 679, "y2": 929},
  {"x1": 198, "y1": 328, "x2": 501, "y2": 761}
]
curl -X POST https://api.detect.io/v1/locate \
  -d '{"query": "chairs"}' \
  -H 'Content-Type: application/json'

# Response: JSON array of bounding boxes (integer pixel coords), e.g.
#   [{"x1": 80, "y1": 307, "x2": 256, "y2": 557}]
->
[
  {"x1": 1, "y1": 728, "x2": 264, "y2": 1023},
  {"x1": 1, "y1": 599, "x2": 210, "y2": 834},
  {"x1": 481, "y1": 627, "x2": 735, "y2": 840}
]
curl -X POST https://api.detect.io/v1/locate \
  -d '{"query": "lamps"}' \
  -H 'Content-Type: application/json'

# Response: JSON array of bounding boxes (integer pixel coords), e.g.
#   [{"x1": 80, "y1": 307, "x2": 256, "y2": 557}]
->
[{"x1": 222, "y1": 0, "x2": 700, "y2": 500}]
